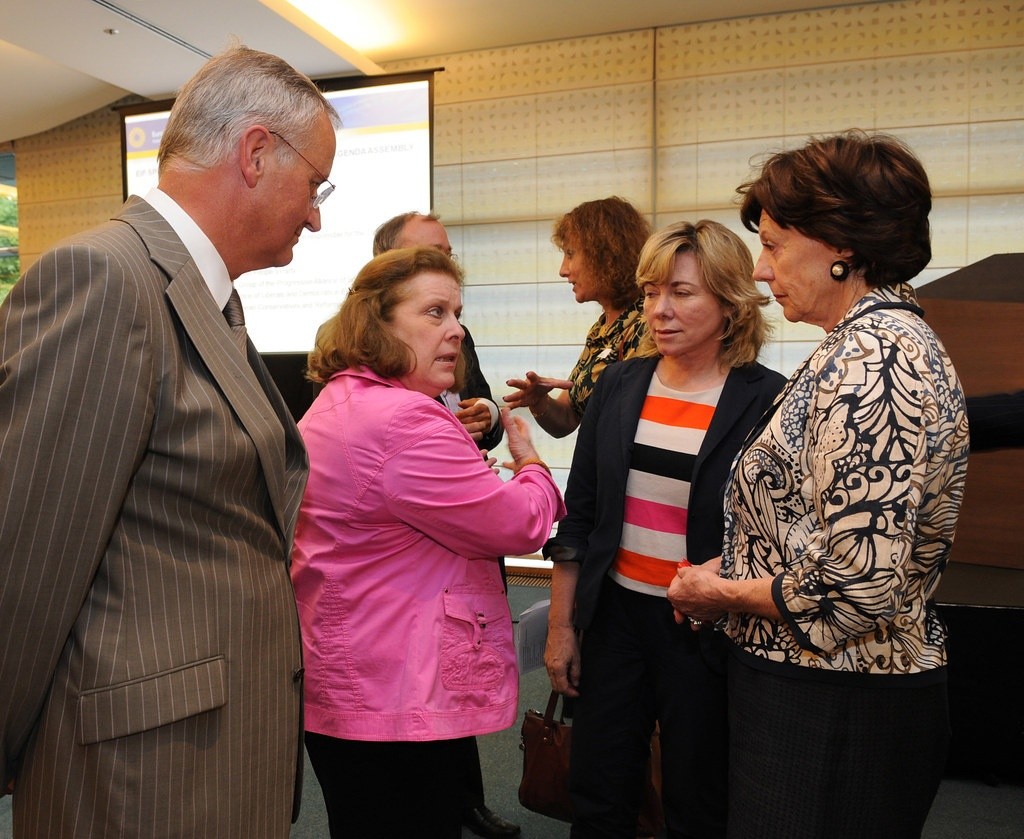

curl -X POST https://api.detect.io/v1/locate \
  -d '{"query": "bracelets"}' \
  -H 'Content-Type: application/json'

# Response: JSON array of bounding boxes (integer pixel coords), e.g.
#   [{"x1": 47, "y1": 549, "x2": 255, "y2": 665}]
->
[{"x1": 515, "y1": 459, "x2": 552, "y2": 476}]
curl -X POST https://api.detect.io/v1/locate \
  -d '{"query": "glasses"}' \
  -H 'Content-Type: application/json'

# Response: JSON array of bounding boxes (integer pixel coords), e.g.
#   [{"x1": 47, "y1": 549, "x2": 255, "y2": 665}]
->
[{"x1": 269, "y1": 130, "x2": 335, "y2": 208}]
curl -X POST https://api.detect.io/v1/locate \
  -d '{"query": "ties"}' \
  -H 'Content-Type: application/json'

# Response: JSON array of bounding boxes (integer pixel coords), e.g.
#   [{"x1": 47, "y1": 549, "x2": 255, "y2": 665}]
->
[{"x1": 224, "y1": 287, "x2": 248, "y2": 361}]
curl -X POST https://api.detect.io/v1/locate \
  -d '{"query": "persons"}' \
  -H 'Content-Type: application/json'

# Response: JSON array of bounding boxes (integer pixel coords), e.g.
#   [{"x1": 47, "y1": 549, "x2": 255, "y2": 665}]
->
[
  {"x1": 503, "y1": 199, "x2": 652, "y2": 438},
  {"x1": 544, "y1": 218, "x2": 788, "y2": 839},
  {"x1": 373, "y1": 212, "x2": 521, "y2": 839},
  {"x1": 667, "y1": 129, "x2": 969, "y2": 839},
  {"x1": 1, "y1": 47, "x2": 342, "y2": 839},
  {"x1": 291, "y1": 247, "x2": 566, "y2": 839}
]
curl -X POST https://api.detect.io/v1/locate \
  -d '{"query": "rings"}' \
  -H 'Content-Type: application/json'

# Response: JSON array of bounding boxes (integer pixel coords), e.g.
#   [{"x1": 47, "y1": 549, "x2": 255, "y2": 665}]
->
[{"x1": 689, "y1": 617, "x2": 702, "y2": 625}]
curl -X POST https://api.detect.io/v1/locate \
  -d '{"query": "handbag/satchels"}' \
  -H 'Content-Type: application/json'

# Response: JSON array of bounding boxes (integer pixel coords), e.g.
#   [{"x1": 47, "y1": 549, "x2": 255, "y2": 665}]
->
[{"x1": 518, "y1": 691, "x2": 665, "y2": 835}]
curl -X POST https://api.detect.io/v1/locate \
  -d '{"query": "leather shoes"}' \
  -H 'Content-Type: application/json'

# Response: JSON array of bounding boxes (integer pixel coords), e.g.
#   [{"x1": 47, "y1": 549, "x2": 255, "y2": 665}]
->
[{"x1": 461, "y1": 805, "x2": 520, "y2": 839}]
